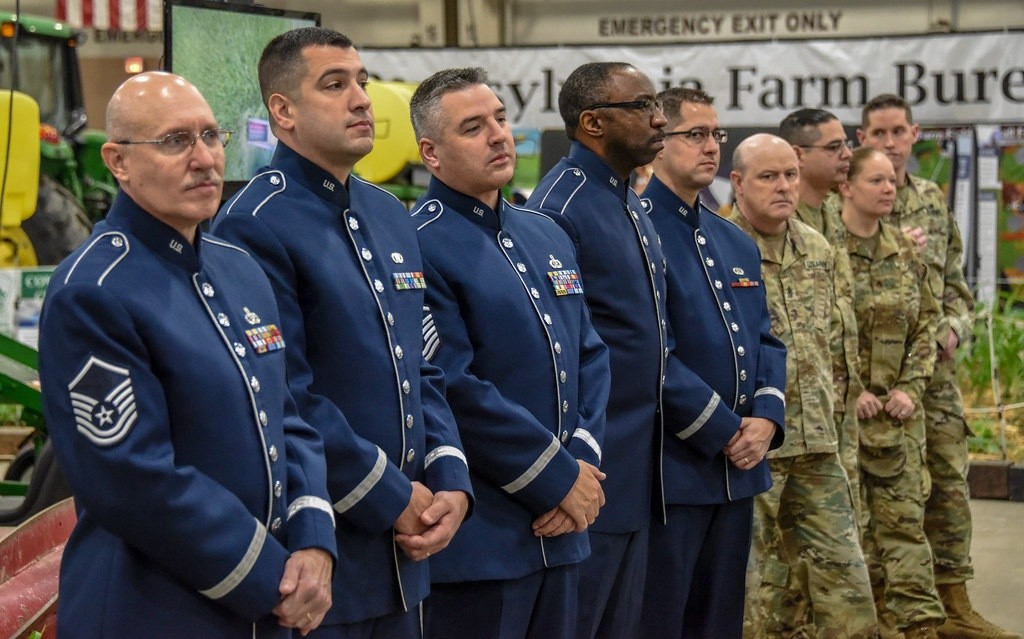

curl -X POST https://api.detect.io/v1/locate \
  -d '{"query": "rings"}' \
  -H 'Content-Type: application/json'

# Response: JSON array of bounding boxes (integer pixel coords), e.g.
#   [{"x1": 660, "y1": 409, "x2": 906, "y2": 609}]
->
[
  {"x1": 307, "y1": 613, "x2": 312, "y2": 621},
  {"x1": 744, "y1": 458, "x2": 749, "y2": 463},
  {"x1": 900, "y1": 411, "x2": 905, "y2": 416},
  {"x1": 426, "y1": 553, "x2": 430, "y2": 557}
]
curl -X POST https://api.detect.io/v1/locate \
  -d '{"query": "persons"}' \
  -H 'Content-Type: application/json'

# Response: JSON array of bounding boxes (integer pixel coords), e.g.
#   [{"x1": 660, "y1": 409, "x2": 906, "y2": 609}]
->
[
  {"x1": 525, "y1": 62, "x2": 669, "y2": 639},
  {"x1": 726, "y1": 93, "x2": 1024, "y2": 639},
  {"x1": 406, "y1": 65, "x2": 612, "y2": 639},
  {"x1": 639, "y1": 89, "x2": 787, "y2": 639},
  {"x1": 209, "y1": 27, "x2": 475, "y2": 639},
  {"x1": 40, "y1": 71, "x2": 338, "y2": 639}
]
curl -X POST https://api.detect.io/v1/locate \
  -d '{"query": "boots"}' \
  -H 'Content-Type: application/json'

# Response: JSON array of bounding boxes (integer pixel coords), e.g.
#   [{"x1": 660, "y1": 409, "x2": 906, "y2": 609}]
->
[
  {"x1": 904, "y1": 619, "x2": 939, "y2": 639},
  {"x1": 935, "y1": 580, "x2": 1024, "y2": 639}
]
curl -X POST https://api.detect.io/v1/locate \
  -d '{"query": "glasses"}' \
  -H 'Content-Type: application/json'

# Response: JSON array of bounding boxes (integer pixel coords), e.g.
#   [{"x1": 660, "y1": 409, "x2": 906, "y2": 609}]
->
[
  {"x1": 585, "y1": 97, "x2": 664, "y2": 115},
  {"x1": 113, "y1": 127, "x2": 234, "y2": 152},
  {"x1": 665, "y1": 129, "x2": 728, "y2": 143},
  {"x1": 798, "y1": 139, "x2": 854, "y2": 153}
]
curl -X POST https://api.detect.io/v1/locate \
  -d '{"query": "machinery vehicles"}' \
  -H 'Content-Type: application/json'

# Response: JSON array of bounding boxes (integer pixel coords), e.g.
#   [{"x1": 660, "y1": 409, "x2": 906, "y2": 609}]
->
[{"x1": 2, "y1": 12, "x2": 121, "y2": 265}]
